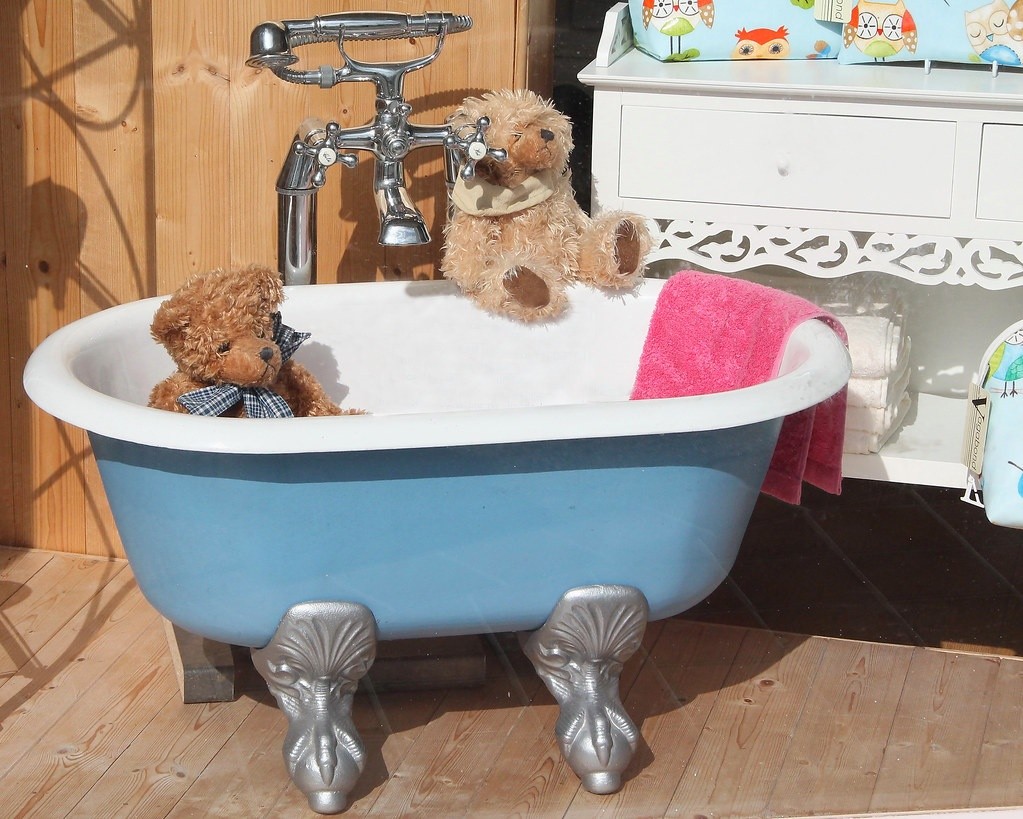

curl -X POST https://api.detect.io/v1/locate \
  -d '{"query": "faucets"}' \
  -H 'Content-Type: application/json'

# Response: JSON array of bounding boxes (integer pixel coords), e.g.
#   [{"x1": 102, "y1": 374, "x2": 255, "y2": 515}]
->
[{"x1": 361, "y1": 107, "x2": 436, "y2": 249}]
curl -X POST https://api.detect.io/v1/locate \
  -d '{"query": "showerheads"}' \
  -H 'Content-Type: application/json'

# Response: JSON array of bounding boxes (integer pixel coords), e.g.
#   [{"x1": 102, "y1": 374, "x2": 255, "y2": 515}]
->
[{"x1": 241, "y1": 9, "x2": 477, "y2": 72}]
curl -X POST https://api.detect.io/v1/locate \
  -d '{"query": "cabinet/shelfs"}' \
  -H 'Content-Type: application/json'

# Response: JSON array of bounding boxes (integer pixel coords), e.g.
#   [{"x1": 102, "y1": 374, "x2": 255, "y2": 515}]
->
[{"x1": 590, "y1": 86, "x2": 1023, "y2": 493}]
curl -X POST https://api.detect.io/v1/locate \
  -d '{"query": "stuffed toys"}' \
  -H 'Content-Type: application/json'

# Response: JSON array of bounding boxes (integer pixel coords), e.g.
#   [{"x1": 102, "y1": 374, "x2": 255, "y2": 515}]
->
[
  {"x1": 148, "y1": 262, "x2": 372, "y2": 419},
  {"x1": 440, "y1": 88, "x2": 655, "y2": 326}
]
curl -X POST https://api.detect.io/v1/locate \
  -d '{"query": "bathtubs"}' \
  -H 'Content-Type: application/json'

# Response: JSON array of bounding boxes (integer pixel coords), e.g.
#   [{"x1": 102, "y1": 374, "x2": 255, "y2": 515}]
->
[{"x1": 21, "y1": 269, "x2": 862, "y2": 819}]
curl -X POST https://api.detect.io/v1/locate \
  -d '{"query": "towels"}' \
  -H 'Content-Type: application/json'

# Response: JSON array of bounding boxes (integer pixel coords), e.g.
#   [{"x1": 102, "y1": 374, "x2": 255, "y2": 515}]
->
[
  {"x1": 740, "y1": 274, "x2": 918, "y2": 460},
  {"x1": 627, "y1": 269, "x2": 854, "y2": 512}
]
